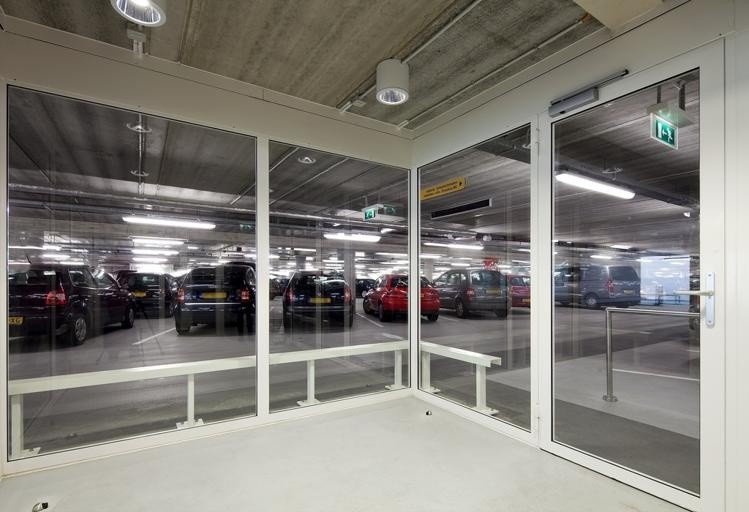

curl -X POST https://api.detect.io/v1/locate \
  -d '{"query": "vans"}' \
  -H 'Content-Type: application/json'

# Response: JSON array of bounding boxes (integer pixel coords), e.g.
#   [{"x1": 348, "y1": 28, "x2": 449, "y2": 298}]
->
[{"x1": 552, "y1": 264, "x2": 642, "y2": 310}]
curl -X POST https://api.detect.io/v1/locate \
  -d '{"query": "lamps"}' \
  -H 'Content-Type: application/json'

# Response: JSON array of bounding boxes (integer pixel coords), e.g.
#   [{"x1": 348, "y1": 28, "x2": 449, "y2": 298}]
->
[
  {"x1": 109, "y1": 0, "x2": 167, "y2": 27},
  {"x1": 376, "y1": 59, "x2": 410, "y2": 105}
]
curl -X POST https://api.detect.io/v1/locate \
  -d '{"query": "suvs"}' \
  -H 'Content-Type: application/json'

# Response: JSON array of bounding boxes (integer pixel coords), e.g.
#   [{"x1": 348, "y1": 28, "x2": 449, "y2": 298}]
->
[
  {"x1": 7, "y1": 259, "x2": 136, "y2": 347},
  {"x1": 172, "y1": 260, "x2": 254, "y2": 335}
]
